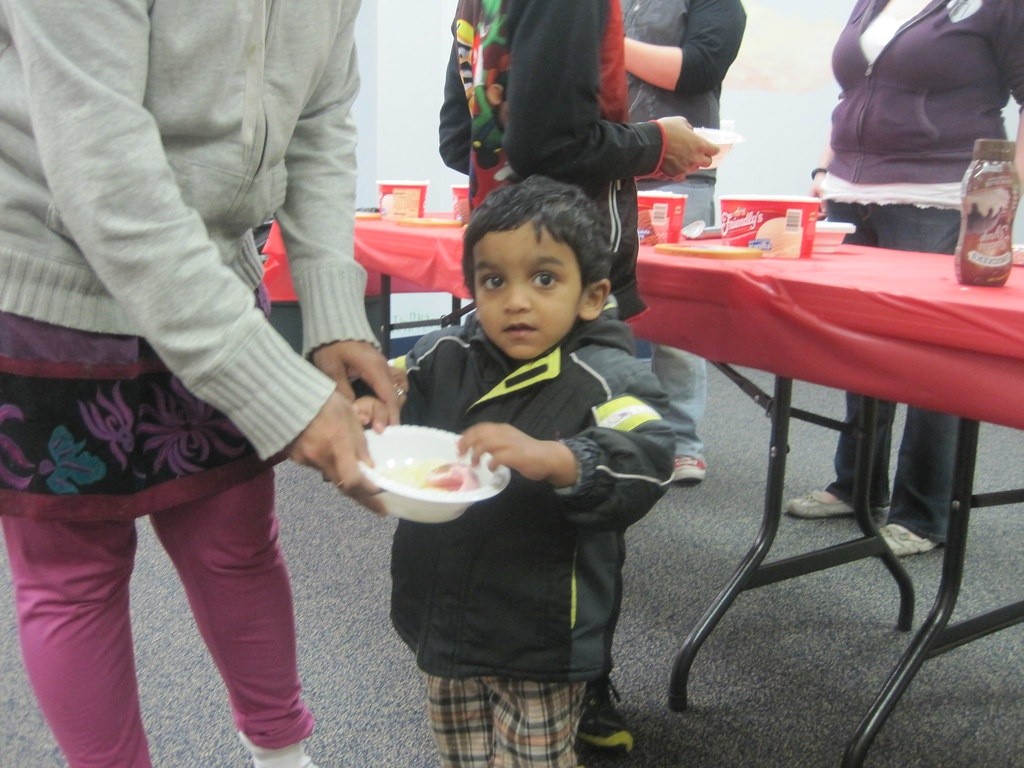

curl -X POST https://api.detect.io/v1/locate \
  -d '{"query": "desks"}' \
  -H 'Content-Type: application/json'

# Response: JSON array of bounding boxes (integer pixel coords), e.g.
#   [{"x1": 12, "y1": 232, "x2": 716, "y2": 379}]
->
[{"x1": 262, "y1": 212, "x2": 1024, "y2": 768}]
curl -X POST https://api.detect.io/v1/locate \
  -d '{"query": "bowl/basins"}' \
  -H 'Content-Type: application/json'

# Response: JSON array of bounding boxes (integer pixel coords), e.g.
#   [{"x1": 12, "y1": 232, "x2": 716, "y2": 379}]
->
[
  {"x1": 691, "y1": 126, "x2": 743, "y2": 171},
  {"x1": 636, "y1": 191, "x2": 688, "y2": 246},
  {"x1": 719, "y1": 196, "x2": 821, "y2": 259},
  {"x1": 378, "y1": 179, "x2": 427, "y2": 219},
  {"x1": 450, "y1": 185, "x2": 470, "y2": 223},
  {"x1": 357, "y1": 423, "x2": 512, "y2": 523},
  {"x1": 814, "y1": 222, "x2": 856, "y2": 246}
]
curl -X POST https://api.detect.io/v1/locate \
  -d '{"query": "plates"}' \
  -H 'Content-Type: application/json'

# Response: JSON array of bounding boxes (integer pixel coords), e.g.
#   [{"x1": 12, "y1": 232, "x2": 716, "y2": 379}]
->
[
  {"x1": 652, "y1": 242, "x2": 763, "y2": 260},
  {"x1": 355, "y1": 211, "x2": 382, "y2": 221},
  {"x1": 398, "y1": 217, "x2": 463, "y2": 229}
]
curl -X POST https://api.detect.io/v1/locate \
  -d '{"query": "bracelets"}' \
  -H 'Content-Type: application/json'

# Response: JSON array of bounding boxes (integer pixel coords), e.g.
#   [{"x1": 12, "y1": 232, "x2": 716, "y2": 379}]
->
[{"x1": 811, "y1": 168, "x2": 828, "y2": 180}]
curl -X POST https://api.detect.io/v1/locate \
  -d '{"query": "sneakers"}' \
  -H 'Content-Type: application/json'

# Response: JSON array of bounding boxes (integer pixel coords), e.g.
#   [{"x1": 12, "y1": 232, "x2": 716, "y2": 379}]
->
[{"x1": 572, "y1": 677, "x2": 633, "y2": 756}]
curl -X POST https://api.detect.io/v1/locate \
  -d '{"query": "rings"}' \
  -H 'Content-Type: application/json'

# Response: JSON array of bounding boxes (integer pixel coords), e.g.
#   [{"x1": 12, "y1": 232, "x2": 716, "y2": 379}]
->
[{"x1": 393, "y1": 382, "x2": 405, "y2": 397}]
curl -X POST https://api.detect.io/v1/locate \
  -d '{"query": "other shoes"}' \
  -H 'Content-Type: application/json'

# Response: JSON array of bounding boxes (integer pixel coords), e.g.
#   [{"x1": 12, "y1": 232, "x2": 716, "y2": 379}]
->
[
  {"x1": 877, "y1": 525, "x2": 939, "y2": 557},
  {"x1": 787, "y1": 490, "x2": 855, "y2": 518},
  {"x1": 673, "y1": 455, "x2": 705, "y2": 482}
]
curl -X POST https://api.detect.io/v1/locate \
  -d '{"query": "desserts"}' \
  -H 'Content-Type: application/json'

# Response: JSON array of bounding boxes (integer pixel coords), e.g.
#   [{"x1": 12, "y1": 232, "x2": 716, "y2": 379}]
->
[
  {"x1": 636, "y1": 208, "x2": 668, "y2": 244},
  {"x1": 756, "y1": 217, "x2": 801, "y2": 258},
  {"x1": 454, "y1": 198, "x2": 470, "y2": 225},
  {"x1": 381, "y1": 193, "x2": 392, "y2": 214},
  {"x1": 378, "y1": 461, "x2": 485, "y2": 493}
]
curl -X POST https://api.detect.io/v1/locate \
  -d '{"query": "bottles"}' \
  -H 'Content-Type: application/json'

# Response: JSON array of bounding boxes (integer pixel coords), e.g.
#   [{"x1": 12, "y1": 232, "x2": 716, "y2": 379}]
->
[{"x1": 954, "y1": 138, "x2": 1020, "y2": 287}]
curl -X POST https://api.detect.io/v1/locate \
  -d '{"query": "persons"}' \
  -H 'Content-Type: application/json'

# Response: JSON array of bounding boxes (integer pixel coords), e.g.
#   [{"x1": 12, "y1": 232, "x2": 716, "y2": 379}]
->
[
  {"x1": 351, "y1": 175, "x2": 676, "y2": 768},
  {"x1": 0, "y1": 1, "x2": 410, "y2": 768},
  {"x1": 439, "y1": 0, "x2": 746, "y2": 485},
  {"x1": 783, "y1": 0, "x2": 1023, "y2": 555}
]
